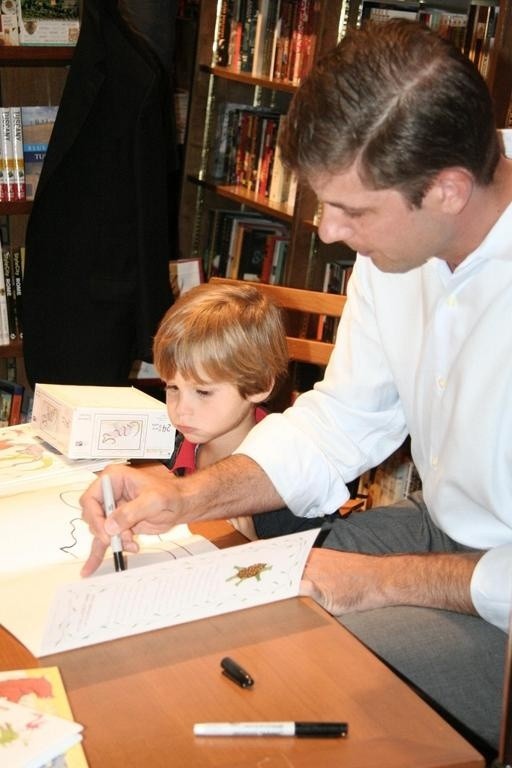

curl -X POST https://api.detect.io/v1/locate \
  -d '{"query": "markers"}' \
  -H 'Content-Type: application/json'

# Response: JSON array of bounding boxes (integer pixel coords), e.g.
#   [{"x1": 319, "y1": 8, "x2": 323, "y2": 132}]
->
[
  {"x1": 102, "y1": 474, "x2": 125, "y2": 571},
  {"x1": 192, "y1": 720, "x2": 348, "y2": 738}
]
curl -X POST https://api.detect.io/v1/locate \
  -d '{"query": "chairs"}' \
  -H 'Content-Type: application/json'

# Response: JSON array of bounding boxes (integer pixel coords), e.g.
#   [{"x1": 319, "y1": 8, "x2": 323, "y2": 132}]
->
[{"x1": 206, "y1": 276, "x2": 348, "y2": 409}]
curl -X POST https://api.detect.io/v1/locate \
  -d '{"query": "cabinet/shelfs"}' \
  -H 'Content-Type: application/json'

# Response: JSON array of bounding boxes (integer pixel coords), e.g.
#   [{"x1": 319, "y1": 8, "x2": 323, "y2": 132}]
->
[
  {"x1": 2, "y1": 0, "x2": 83, "y2": 425},
  {"x1": 177, "y1": 1, "x2": 512, "y2": 414}
]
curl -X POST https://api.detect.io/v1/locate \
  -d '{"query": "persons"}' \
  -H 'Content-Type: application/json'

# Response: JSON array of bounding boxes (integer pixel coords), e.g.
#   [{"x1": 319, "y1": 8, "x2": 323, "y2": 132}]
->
[
  {"x1": 80, "y1": 17, "x2": 512, "y2": 757},
  {"x1": 151, "y1": 278, "x2": 343, "y2": 541}
]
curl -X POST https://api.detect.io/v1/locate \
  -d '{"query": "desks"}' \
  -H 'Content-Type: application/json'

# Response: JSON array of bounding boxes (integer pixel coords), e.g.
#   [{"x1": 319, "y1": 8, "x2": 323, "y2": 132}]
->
[{"x1": 0, "y1": 456, "x2": 489, "y2": 768}]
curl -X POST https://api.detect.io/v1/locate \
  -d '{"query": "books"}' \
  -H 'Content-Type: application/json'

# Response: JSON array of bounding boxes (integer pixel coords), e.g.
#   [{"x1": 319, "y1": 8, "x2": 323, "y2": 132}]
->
[
  {"x1": 219, "y1": 0, "x2": 501, "y2": 91},
  {"x1": 0, "y1": 662, "x2": 90, "y2": 767},
  {"x1": 211, "y1": 98, "x2": 324, "y2": 226},
  {"x1": 1, "y1": 0, "x2": 84, "y2": 427},
  {"x1": 209, "y1": 211, "x2": 423, "y2": 513}
]
